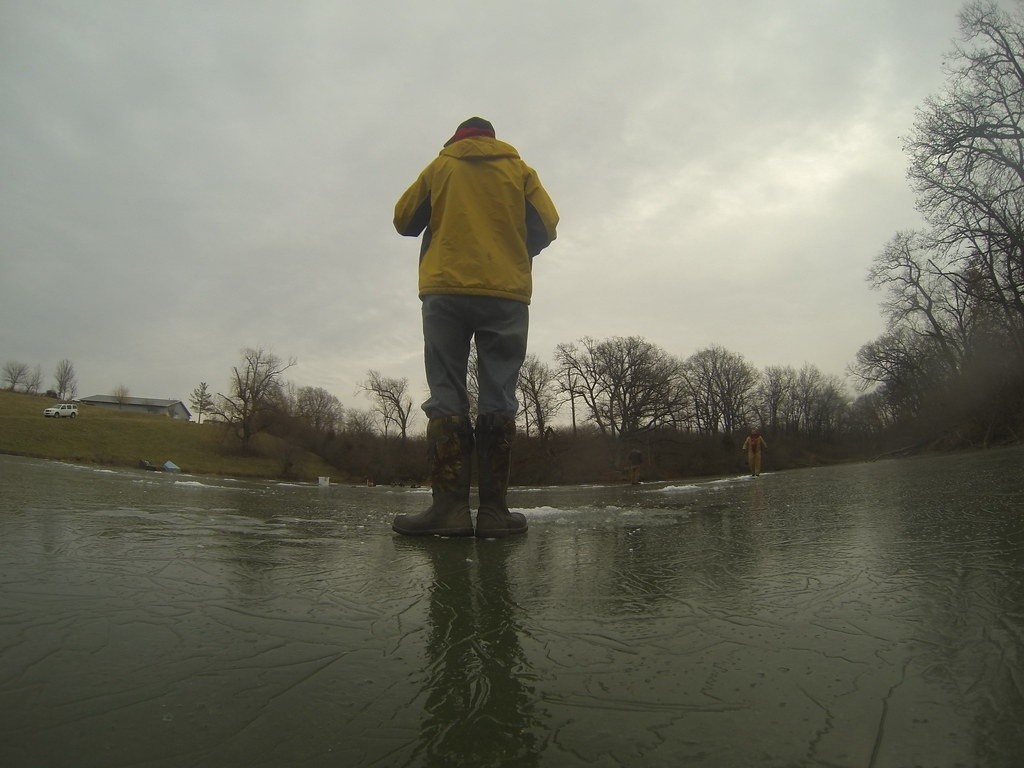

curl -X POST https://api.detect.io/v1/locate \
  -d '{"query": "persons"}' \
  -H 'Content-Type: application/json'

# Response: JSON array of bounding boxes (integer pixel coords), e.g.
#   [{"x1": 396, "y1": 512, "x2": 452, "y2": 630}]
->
[
  {"x1": 743, "y1": 428, "x2": 768, "y2": 478},
  {"x1": 392, "y1": 114, "x2": 560, "y2": 544},
  {"x1": 628, "y1": 445, "x2": 645, "y2": 486}
]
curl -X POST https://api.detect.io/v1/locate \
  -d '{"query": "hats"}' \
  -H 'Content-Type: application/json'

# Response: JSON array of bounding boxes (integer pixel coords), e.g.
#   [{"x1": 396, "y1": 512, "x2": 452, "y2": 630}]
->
[{"x1": 442, "y1": 116, "x2": 496, "y2": 149}]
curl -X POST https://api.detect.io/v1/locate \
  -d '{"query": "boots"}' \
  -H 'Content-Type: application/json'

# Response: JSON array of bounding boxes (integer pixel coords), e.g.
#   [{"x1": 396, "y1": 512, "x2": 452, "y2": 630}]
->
[
  {"x1": 471, "y1": 413, "x2": 528, "y2": 538},
  {"x1": 387, "y1": 415, "x2": 474, "y2": 537}
]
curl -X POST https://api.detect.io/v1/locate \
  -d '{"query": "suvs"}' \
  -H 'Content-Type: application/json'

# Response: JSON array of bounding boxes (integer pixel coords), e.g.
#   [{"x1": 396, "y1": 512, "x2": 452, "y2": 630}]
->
[{"x1": 43, "y1": 403, "x2": 80, "y2": 419}]
